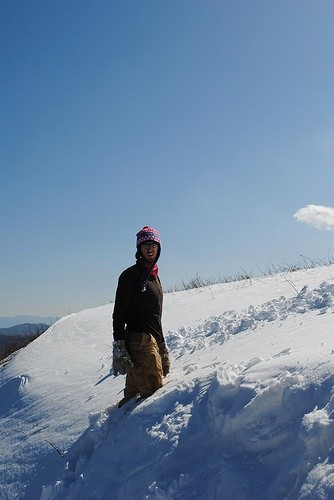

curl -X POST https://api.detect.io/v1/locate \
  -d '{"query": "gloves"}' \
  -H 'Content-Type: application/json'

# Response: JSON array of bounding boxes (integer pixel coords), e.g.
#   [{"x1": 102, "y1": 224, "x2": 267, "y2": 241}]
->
[
  {"x1": 112, "y1": 340, "x2": 132, "y2": 376},
  {"x1": 159, "y1": 342, "x2": 170, "y2": 376}
]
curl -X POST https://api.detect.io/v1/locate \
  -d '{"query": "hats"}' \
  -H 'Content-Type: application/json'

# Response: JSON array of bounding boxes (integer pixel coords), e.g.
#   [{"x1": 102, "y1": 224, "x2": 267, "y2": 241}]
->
[{"x1": 136, "y1": 226, "x2": 160, "y2": 246}]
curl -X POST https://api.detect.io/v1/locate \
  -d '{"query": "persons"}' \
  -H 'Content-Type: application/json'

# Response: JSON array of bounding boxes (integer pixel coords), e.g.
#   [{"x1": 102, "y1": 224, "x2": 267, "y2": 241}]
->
[{"x1": 109, "y1": 225, "x2": 174, "y2": 405}]
